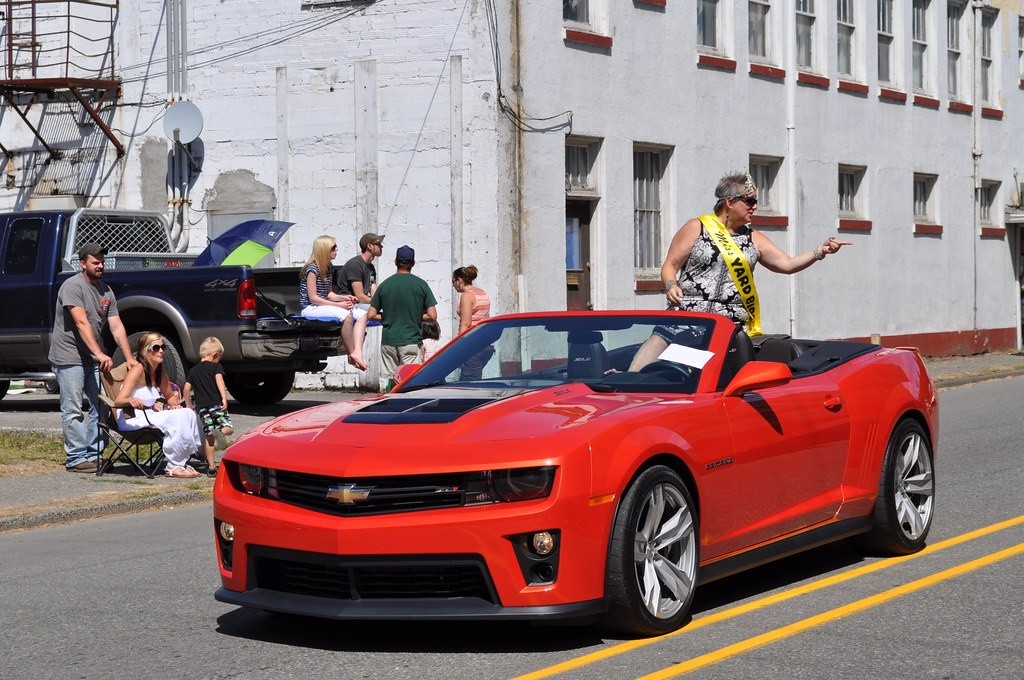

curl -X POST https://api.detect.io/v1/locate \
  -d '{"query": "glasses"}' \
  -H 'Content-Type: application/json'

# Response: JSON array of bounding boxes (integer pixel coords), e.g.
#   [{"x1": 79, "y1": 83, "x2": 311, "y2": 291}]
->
[
  {"x1": 148, "y1": 343, "x2": 168, "y2": 354},
  {"x1": 726, "y1": 195, "x2": 758, "y2": 207},
  {"x1": 452, "y1": 279, "x2": 458, "y2": 284},
  {"x1": 331, "y1": 244, "x2": 338, "y2": 252}
]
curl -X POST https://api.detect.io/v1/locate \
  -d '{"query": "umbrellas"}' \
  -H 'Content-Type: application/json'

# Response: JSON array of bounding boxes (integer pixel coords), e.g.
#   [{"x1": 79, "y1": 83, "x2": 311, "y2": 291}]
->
[{"x1": 192, "y1": 219, "x2": 296, "y2": 326}]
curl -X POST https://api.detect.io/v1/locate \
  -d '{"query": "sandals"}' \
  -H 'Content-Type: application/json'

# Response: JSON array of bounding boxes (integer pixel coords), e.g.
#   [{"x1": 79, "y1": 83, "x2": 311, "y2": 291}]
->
[
  {"x1": 163, "y1": 466, "x2": 195, "y2": 479},
  {"x1": 185, "y1": 465, "x2": 201, "y2": 477}
]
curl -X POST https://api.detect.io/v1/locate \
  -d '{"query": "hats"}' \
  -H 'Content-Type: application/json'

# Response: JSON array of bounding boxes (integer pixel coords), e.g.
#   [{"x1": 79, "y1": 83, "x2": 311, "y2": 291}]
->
[
  {"x1": 78, "y1": 243, "x2": 109, "y2": 259},
  {"x1": 396, "y1": 244, "x2": 415, "y2": 263},
  {"x1": 360, "y1": 232, "x2": 386, "y2": 248}
]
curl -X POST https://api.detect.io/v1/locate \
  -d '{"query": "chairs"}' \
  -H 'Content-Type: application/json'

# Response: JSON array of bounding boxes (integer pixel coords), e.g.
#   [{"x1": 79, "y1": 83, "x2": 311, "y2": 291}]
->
[
  {"x1": 699, "y1": 329, "x2": 753, "y2": 383},
  {"x1": 94, "y1": 358, "x2": 195, "y2": 479},
  {"x1": 567, "y1": 339, "x2": 608, "y2": 378}
]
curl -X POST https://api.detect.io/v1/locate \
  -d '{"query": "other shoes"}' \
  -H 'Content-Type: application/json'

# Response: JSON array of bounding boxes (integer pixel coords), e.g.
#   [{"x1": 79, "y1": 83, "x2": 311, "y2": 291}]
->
[
  {"x1": 66, "y1": 460, "x2": 100, "y2": 473},
  {"x1": 89, "y1": 457, "x2": 114, "y2": 471},
  {"x1": 212, "y1": 428, "x2": 227, "y2": 450},
  {"x1": 206, "y1": 467, "x2": 218, "y2": 477}
]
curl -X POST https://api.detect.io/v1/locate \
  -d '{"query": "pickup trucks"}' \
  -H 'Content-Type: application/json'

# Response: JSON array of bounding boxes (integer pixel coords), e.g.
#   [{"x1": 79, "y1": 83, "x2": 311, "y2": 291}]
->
[{"x1": 0, "y1": 205, "x2": 439, "y2": 410}]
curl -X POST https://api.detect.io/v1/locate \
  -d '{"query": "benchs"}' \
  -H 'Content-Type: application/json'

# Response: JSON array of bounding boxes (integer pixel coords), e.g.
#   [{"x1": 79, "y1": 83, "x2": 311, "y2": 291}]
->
[{"x1": 750, "y1": 338, "x2": 810, "y2": 375}]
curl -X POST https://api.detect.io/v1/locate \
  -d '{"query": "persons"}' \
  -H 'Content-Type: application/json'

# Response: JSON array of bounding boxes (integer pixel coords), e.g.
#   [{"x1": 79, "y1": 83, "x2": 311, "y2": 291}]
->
[
  {"x1": 297, "y1": 235, "x2": 369, "y2": 371},
  {"x1": 183, "y1": 336, "x2": 235, "y2": 477},
  {"x1": 625, "y1": 169, "x2": 853, "y2": 374},
  {"x1": 451, "y1": 264, "x2": 495, "y2": 380},
  {"x1": 48, "y1": 242, "x2": 139, "y2": 473},
  {"x1": 113, "y1": 332, "x2": 203, "y2": 478},
  {"x1": 336, "y1": 233, "x2": 386, "y2": 311},
  {"x1": 418, "y1": 318, "x2": 442, "y2": 363},
  {"x1": 366, "y1": 244, "x2": 438, "y2": 381}
]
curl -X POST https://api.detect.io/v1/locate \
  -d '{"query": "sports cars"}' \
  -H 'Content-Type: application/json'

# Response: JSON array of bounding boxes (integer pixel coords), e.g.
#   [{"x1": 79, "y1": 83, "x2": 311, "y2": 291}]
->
[{"x1": 210, "y1": 304, "x2": 947, "y2": 640}]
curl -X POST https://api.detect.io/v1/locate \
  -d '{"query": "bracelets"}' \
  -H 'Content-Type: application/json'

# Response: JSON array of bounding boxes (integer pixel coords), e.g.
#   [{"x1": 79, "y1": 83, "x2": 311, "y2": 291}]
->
[
  {"x1": 812, "y1": 247, "x2": 826, "y2": 260},
  {"x1": 664, "y1": 280, "x2": 677, "y2": 292}
]
